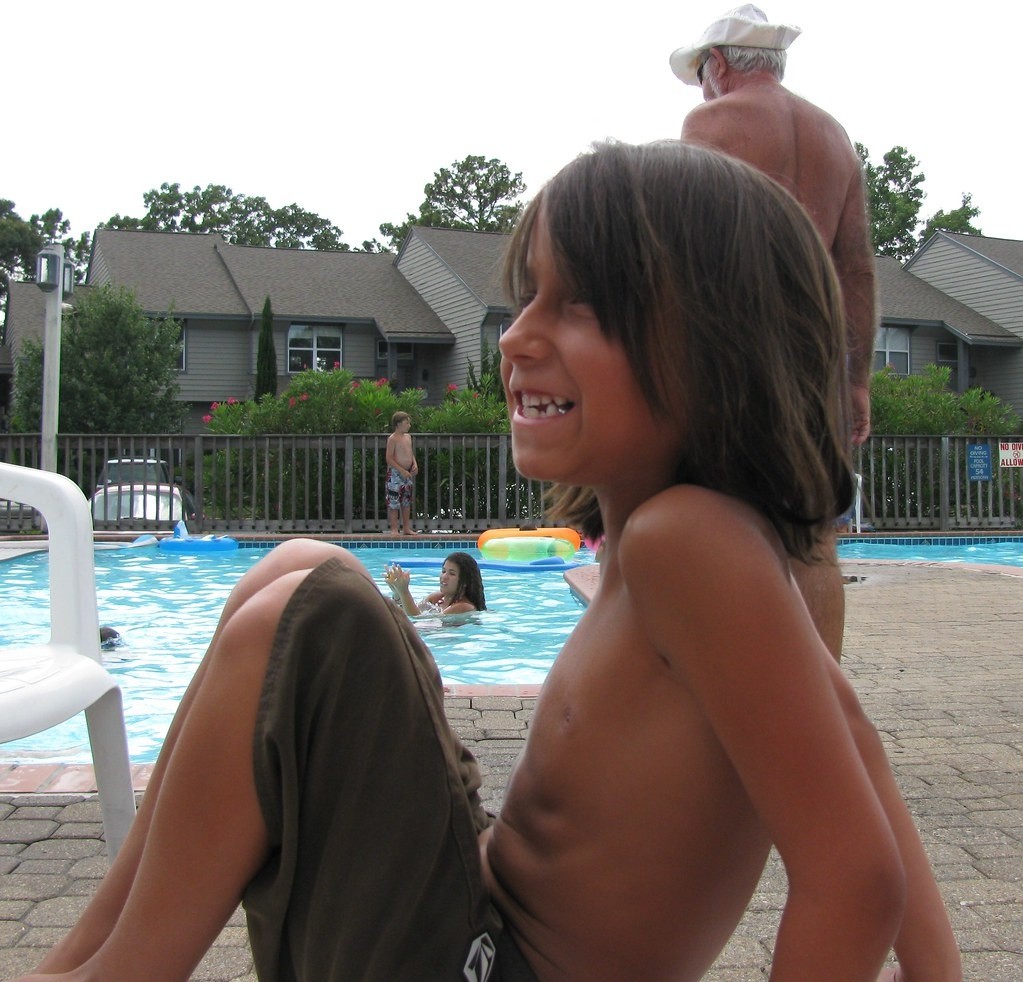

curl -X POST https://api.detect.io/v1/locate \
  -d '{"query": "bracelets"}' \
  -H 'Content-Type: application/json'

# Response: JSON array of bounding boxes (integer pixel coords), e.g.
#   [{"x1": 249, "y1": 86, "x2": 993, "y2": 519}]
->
[{"x1": 393, "y1": 598, "x2": 400, "y2": 602}]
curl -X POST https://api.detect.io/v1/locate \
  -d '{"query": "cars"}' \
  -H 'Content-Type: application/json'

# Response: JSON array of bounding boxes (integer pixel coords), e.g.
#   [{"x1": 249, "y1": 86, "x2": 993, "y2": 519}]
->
[{"x1": 87, "y1": 483, "x2": 210, "y2": 520}]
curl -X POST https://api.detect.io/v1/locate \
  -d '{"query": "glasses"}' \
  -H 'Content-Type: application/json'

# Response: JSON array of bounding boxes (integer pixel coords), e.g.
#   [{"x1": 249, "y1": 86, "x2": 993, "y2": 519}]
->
[{"x1": 696, "y1": 56, "x2": 711, "y2": 86}]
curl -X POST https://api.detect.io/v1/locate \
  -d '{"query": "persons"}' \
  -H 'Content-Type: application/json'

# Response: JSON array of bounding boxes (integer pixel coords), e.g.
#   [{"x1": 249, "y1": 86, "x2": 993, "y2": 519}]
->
[
  {"x1": 386, "y1": 411, "x2": 421, "y2": 535},
  {"x1": 6, "y1": 139, "x2": 964, "y2": 982},
  {"x1": 98, "y1": 629, "x2": 123, "y2": 650},
  {"x1": 382, "y1": 553, "x2": 487, "y2": 617},
  {"x1": 668, "y1": 2, "x2": 879, "y2": 666}
]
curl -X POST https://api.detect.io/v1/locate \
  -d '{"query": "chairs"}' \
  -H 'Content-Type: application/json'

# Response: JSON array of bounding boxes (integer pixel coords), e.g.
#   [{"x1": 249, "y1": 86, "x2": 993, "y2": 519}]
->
[{"x1": 0, "y1": 459, "x2": 138, "y2": 868}]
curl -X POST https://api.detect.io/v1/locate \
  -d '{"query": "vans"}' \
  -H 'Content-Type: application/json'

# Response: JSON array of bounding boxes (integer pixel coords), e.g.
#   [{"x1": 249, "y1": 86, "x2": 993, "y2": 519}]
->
[{"x1": 92, "y1": 457, "x2": 171, "y2": 493}]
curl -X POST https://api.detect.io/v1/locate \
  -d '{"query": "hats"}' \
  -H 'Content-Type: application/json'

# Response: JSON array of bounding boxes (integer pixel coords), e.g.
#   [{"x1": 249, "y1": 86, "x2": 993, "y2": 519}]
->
[{"x1": 669, "y1": 3, "x2": 802, "y2": 87}]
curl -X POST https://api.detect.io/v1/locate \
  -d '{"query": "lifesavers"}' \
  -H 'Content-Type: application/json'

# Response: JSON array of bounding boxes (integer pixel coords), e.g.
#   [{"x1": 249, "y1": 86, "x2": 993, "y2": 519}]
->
[
  {"x1": 482, "y1": 536, "x2": 575, "y2": 562},
  {"x1": 585, "y1": 533, "x2": 606, "y2": 551},
  {"x1": 159, "y1": 520, "x2": 239, "y2": 552},
  {"x1": 478, "y1": 525, "x2": 581, "y2": 555}
]
